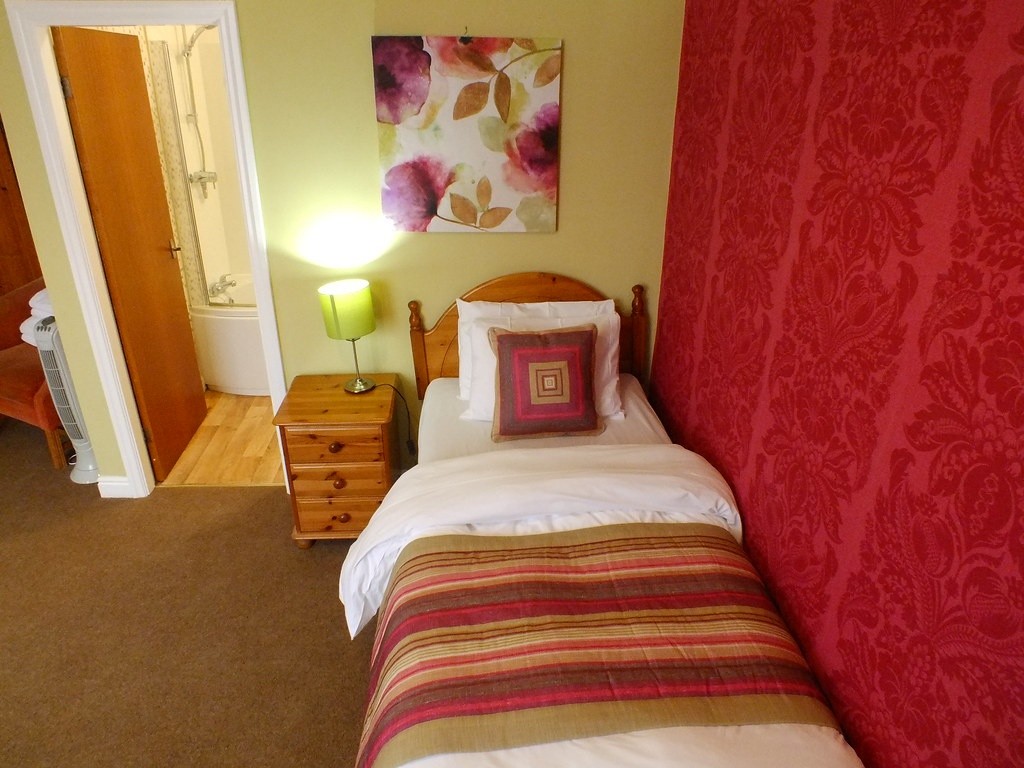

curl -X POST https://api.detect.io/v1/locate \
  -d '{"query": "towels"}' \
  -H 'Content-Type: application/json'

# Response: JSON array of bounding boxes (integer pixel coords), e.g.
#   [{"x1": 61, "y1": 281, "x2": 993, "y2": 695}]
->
[{"x1": 19, "y1": 284, "x2": 57, "y2": 352}]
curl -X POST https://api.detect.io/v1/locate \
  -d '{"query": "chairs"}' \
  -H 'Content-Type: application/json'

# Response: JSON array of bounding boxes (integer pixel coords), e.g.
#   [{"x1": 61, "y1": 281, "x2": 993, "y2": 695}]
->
[{"x1": 0, "y1": 275, "x2": 72, "y2": 470}]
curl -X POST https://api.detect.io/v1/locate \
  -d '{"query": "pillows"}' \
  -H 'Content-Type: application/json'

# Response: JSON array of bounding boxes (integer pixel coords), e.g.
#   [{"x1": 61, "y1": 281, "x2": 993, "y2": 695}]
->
[
  {"x1": 484, "y1": 321, "x2": 609, "y2": 447},
  {"x1": 464, "y1": 311, "x2": 627, "y2": 422},
  {"x1": 452, "y1": 298, "x2": 620, "y2": 401}
]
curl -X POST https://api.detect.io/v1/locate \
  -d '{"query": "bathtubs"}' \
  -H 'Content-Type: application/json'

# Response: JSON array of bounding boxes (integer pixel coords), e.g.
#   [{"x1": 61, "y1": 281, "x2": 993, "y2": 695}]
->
[{"x1": 186, "y1": 270, "x2": 272, "y2": 399}]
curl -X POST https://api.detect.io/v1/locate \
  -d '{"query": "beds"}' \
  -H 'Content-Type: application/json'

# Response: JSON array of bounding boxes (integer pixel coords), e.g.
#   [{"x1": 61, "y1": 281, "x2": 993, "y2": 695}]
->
[{"x1": 335, "y1": 272, "x2": 872, "y2": 766}]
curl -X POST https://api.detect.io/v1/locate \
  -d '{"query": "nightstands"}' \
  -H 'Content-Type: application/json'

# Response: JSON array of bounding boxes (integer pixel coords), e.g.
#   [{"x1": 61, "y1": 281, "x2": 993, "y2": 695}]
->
[{"x1": 272, "y1": 373, "x2": 399, "y2": 550}]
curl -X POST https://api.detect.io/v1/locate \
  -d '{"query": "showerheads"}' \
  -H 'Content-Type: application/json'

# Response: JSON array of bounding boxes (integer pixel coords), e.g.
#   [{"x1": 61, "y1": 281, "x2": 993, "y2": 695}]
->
[{"x1": 185, "y1": 24, "x2": 217, "y2": 51}]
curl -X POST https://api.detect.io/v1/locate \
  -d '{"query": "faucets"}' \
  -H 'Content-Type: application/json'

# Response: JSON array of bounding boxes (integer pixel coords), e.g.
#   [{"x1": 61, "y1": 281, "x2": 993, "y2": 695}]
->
[{"x1": 211, "y1": 273, "x2": 240, "y2": 297}]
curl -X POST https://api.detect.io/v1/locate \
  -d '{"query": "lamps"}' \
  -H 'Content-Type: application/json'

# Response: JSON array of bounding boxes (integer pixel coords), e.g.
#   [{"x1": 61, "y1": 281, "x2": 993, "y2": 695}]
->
[{"x1": 317, "y1": 279, "x2": 380, "y2": 395}]
